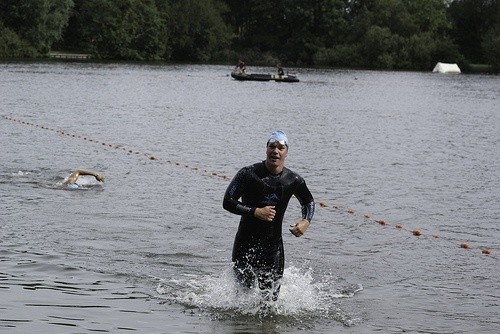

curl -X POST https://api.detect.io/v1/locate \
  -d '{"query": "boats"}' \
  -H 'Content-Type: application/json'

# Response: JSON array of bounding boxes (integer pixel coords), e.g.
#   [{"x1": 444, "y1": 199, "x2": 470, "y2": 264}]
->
[{"x1": 230, "y1": 70, "x2": 300, "y2": 83}]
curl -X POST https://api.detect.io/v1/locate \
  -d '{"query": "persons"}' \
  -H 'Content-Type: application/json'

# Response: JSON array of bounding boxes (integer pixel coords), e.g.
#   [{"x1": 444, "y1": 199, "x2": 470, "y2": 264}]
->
[
  {"x1": 235, "y1": 59, "x2": 246, "y2": 74},
  {"x1": 222, "y1": 131, "x2": 315, "y2": 306},
  {"x1": 278, "y1": 66, "x2": 284, "y2": 79}
]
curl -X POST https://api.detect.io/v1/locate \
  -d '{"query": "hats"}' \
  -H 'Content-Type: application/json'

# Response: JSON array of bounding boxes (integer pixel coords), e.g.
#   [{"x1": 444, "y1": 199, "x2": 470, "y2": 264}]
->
[{"x1": 267, "y1": 130, "x2": 289, "y2": 151}]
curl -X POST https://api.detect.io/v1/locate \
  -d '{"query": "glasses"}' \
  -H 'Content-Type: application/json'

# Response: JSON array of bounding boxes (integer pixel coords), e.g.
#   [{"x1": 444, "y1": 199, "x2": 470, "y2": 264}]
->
[{"x1": 270, "y1": 139, "x2": 285, "y2": 146}]
